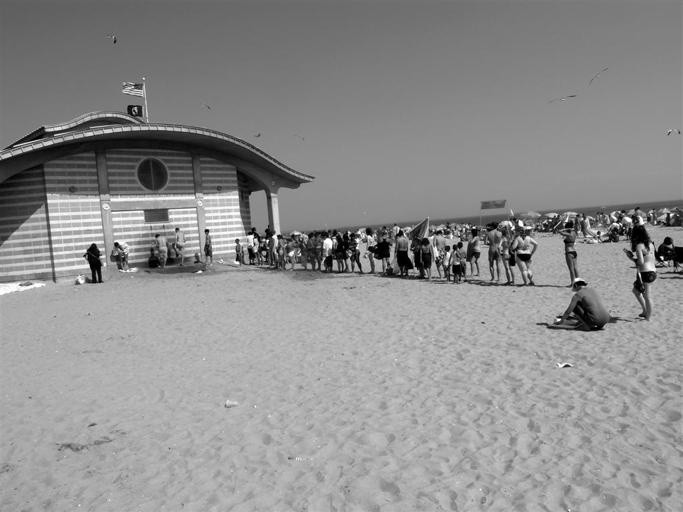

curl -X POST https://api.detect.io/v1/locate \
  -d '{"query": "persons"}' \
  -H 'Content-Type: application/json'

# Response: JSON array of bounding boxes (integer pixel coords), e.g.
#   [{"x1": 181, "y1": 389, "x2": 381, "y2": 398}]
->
[
  {"x1": 623, "y1": 223, "x2": 657, "y2": 320},
  {"x1": 147, "y1": 252, "x2": 161, "y2": 269},
  {"x1": 153, "y1": 233, "x2": 169, "y2": 269},
  {"x1": 363, "y1": 202, "x2": 683, "y2": 290},
  {"x1": 203, "y1": 228, "x2": 213, "y2": 268},
  {"x1": 111, "y1": 240, "x2": 130, "y2": 272},
  {"x1": 82, "y1": 244, "x2": 103, "y2": 283},
  {"x1": 234, "y1": 238, "x2": 242, "y2": 266},
  {"x1": 552, "y1": 277, "x2": 610, "y2": 332},
  {"x1": 170, "y1": 226, "x2": 184, "y2": 267},
  {"x1": 246, "y1": 224, "x2": 365, "y2": 273}
]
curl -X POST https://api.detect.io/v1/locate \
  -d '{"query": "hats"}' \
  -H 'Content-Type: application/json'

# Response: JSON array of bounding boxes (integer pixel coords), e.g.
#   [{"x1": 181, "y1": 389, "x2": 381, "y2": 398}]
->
[{"x1": 572, "y1": 277, "x2": 588, "y2": 291}]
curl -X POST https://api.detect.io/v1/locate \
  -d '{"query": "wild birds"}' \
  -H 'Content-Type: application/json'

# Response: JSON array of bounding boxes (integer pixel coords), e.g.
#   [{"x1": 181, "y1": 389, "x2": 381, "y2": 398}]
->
[
  {"x1": 665, "y1": 127, "x2": 680, "y2": 136},
  {"x1": 586, "y1": 66, "x2": 609, "y2": 88},
  {"x1": 549, "y1": 94, "x2": 576, "y2": 103}
]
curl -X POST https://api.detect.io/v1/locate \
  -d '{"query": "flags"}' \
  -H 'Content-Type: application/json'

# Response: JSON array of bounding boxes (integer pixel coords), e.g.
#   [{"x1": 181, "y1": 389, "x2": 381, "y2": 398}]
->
[
  {"x1": 125, "y1": 105, "x2": 145, "y2": 117},
  {"x1": 120, "y1": 80, "x2": 145, "y2": 97}
]
